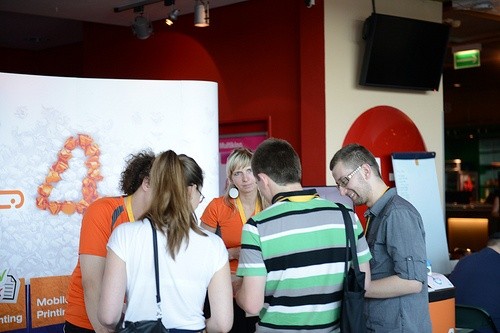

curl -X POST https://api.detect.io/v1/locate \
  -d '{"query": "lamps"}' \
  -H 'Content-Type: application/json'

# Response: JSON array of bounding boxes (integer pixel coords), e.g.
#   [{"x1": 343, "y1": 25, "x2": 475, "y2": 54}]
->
[
  {"x1": 130, "y1": 14, "x2": 153, "y2": 40},
  {"x1": 165, "y1": 8, "x2": 180, "y2": 26},
  {"x1": 193, "y1": 0, "x2": 210, "y2": 27}
]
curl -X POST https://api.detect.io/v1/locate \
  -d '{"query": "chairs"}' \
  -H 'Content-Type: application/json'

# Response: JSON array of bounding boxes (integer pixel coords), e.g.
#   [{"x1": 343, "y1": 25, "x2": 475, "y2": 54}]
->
[{"x1": 455, "y1": 304, "x2": 500, "y2": 333}]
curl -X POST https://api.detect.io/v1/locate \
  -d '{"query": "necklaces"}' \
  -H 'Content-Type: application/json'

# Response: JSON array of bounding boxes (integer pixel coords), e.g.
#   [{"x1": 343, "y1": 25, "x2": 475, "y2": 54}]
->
[
  {"x1": 126, "y1": 196, "x2": 136, "y2": 224},
  {"x1": 235, "y1": 196, "x2": 263, "y2": 227}
]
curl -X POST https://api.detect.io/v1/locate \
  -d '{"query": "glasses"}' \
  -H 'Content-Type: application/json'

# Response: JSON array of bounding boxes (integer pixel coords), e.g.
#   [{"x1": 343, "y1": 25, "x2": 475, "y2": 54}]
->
[
  {"x1": 336, "y1": 163, "x2": 364, "y2": 190},
  {"x1": 195, "y1": 187, "x2": 204, "y2": 203}
]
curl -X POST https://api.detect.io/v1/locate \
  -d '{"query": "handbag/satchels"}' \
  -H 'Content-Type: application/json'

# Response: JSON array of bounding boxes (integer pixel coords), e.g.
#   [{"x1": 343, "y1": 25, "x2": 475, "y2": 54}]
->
[
  {"x1": 340, "y1": 267, "x2": 366, "y2": 333},
  {"x1": 115, "y1": 317, "x2": 168, "y2": 333}
]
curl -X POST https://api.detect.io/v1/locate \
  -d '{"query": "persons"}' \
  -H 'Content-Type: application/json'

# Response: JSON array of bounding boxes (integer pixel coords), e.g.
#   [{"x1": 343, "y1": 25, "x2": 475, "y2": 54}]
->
[
  {"x1": 61, "y1": 150, "x2": 159, "y2": 333},
  {"x1": 96, "y1": 149, "x2": 234, "y2": 333},
  {"x1": 330, "y1": 141, "x2": 433, "y2": 333},
  {"x1": 231, "y1": 137, "x2": 373, "y2": 332},
  {"x1": 449, "y1": 213, "x2": 500, "y2": 332},
  {"x1": 203, "y1": 145, "x2": 271, "y2": 333}
]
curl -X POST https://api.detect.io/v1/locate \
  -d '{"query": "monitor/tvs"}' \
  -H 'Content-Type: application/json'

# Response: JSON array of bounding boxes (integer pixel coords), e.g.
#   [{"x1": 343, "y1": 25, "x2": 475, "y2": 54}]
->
[{"x1": 359, "y1": 14, "x2": 452, "y2": 93}]
